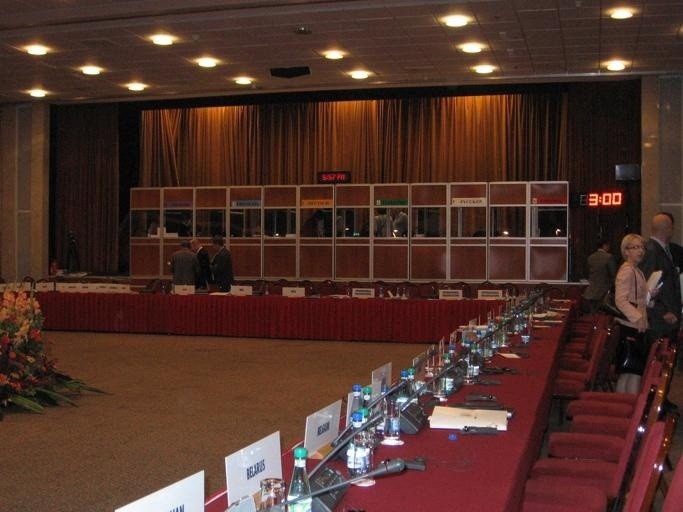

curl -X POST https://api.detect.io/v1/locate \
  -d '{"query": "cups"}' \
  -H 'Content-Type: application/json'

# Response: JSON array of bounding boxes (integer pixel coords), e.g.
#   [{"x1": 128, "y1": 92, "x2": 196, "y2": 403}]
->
[{"x1": 259, "y1": 477, "x2": 286, "y2": 512}]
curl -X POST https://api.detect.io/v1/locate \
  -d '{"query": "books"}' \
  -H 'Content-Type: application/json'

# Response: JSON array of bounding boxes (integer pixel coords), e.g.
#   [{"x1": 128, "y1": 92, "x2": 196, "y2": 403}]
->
[{"x1": 644, "y1": 269, "x2": 661, "y2": 305}]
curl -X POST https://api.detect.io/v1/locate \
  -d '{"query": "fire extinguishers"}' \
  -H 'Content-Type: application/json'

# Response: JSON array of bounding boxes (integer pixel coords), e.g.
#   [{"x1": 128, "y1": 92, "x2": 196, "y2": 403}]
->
[{"x1": 50, "y1": 257, "x2": 60, "y2": 274}]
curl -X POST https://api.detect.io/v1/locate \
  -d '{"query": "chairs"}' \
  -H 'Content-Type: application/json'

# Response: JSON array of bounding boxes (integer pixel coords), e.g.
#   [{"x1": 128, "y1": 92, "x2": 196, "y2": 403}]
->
[
  {"x1": 1, "y1": 276, "x2": 617, "y2": 343},
  {"x1": 522, "y1": 344, "x2": 682, "y2": 511}
]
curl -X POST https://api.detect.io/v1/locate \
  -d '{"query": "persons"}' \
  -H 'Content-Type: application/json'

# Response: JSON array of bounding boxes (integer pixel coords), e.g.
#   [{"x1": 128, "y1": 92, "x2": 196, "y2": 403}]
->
[
  {"x1": 580, "y1": 235, "x2": 616, "y2": 305},
  {"x1": 634, "y1": 214, "x2": 682, "y2": 343},
  {"x1": 209, "y1": 236, "x2": 233, "y2": 290},
  {"x1": 169, "y1": 242, "x2": 199, "y2": 289},
  {"x1": 140, "y1": 208, "x2": 410, "y2": 237},
  {"x1": 603, "y1": 233, "x2": 663, "y2": 393},
  {"x1": 657, "y1": 211, "x2": 682, "y2": 279},
  {"x1": 189, "y1": 239, "x2": 211, "y2": 288}
]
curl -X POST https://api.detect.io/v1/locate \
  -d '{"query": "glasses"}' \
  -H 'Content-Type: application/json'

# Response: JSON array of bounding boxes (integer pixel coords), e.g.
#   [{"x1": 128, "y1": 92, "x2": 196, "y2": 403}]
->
[{"x1": 627, "y1": 245, "x2": 645, "y2": 249}]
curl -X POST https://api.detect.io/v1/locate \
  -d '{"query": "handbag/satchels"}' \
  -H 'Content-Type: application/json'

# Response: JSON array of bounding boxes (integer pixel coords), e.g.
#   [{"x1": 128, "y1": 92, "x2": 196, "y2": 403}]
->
[{"x1": 601, "y1": 290, "x2": 638, "y2": 320}]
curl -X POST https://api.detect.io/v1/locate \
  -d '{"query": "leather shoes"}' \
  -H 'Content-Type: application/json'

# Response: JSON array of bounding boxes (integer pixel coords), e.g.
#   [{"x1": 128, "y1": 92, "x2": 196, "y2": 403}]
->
[{"x1": 665, "y1": 397, "x2": 677, "y2": 409}]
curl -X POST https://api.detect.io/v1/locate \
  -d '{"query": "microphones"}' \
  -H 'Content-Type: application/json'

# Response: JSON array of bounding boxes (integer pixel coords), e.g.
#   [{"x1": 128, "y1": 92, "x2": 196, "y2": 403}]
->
[
  {"x1": 399, "y1": 293, "x2": 544, "y2": 436},
  {"x1": 308, "y1": 410, "x2": 385, "y2": 512},
  {"x1": 309, "y1": 281, "x2": 439, "y2": 300},
  {"x1": 462, "y1": 285, "x2": 472, "y2": 300},
  {"x1": 331, "y1": 382, "x2": 404, "y2": 461},
  {"x1": 281, "y1": 457, "x2": 405, "y2": 509}
]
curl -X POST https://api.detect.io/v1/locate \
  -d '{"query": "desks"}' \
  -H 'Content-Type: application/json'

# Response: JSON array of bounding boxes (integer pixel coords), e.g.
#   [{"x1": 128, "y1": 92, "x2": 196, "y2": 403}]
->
[{"x1": 202, "y1": 344, "x2": 560, "y2": 511}]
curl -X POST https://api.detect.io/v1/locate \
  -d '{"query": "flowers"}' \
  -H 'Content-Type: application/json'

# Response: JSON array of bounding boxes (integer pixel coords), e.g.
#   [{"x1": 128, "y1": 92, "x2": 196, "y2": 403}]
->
[{"x1": 1, "y1": 290, "x2": 112, "y2": 411}]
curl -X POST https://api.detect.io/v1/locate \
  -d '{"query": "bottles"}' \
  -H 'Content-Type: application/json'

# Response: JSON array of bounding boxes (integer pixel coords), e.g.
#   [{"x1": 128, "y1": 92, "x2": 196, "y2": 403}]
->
[
  {"x1": 431, "y1": 312, "x2": 531, "y2": 399},
  {"x1": 286, "y1": 446, "x2": 313, "y2": 512},
  {"x1": 399, "y1": 367, "x2": 418, "y2": 404},
  {"x1": 346, "y1": 383, "x2": 400, "y2": 478}
]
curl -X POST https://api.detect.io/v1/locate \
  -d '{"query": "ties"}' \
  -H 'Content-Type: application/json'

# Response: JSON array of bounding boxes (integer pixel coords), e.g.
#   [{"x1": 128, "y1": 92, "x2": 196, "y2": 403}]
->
[{"x1": 665, "y1": 246, "x2": 669, "y2": 256}]
